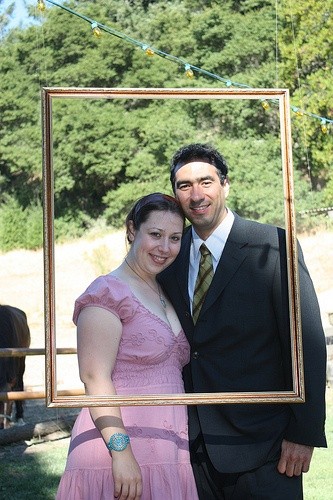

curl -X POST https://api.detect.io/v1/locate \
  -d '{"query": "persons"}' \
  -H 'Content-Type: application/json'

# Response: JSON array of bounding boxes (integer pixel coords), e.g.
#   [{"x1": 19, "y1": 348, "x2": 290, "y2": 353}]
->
[
  {"x1": 55, "y1": 192, "x2": 198, "y2": 500},
  {"x1": 155, "y1": 143, "x2": 329, "y2": 500}
]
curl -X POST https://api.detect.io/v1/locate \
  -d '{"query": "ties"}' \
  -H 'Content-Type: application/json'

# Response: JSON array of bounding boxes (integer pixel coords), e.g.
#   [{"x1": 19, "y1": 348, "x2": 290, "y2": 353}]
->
[{"x1": 191, "y1": 243, "x2": 215, "y2": 326}]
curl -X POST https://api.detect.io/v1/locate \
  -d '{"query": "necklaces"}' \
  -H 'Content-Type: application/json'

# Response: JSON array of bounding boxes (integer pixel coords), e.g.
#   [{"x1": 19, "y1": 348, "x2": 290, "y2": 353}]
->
[{"x1": 125, "y1": 259, "x2": 166, "y2": 307}]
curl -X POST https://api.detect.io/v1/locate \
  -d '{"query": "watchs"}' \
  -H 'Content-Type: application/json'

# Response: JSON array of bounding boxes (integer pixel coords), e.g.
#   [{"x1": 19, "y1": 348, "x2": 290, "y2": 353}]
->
[{"x1": 106, "y1": 432, "x2": 130, "y2": 452}]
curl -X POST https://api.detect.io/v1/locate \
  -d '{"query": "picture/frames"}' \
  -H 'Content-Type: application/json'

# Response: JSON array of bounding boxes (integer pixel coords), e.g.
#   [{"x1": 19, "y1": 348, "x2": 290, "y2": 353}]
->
[{"x1": 42, "y1": 87, "x2": 306, "y2": 409}]
